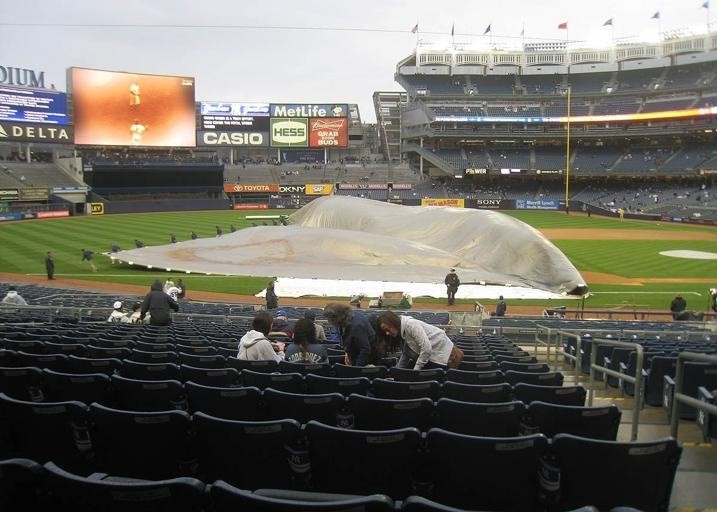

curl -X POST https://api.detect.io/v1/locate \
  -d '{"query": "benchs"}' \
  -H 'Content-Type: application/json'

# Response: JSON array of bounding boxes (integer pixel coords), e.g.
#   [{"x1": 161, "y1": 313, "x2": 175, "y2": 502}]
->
[
  {"x1": 0, "y1": 160, "x2": 84, "y2": 191},
  {"x1": 222, "y1": 159, "x2": 421, "y2": 184}
]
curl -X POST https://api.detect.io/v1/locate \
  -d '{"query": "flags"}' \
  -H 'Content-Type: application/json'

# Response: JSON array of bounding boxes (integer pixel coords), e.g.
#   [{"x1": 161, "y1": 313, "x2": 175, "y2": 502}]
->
[
  {"x1": 519, "y1": 30, "x2": 523, "y2": 36},
  {"x1": 557, "y1": 22, "x2": 567, "y2": 30},
  {"x1": 450, "y1": 23, "x2": 454, "y2": 37},
  {"x1": 601, "y1": 18, "x2": 612, "y2": 27},
  {"x1": 649, "y1": 11, "x2": 659, "y2": 19},
  {"x1": 410, "y1": 24, "x2": 418, "y2": 34},
  {"x1": 701, "y1": 0, "x2": 709, "y2": 9},
  {"x1": 482, "y1": 25, "x2": 490, "y2": 35}
]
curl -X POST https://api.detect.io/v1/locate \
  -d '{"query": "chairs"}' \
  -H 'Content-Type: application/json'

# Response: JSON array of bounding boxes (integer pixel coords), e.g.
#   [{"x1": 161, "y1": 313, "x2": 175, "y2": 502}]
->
[
  {"x1": 569, "y1": 181, "x2": 716, "y2": 219},
  {"x1": 336, "y1": 176, "x2": 571, "y2": 202},
  {"x1": 430, "y1": 97, "x2": 717, "y2": 115},
  {"x1": 428, "y1": 147, "x2": 716, "y2": 171}
]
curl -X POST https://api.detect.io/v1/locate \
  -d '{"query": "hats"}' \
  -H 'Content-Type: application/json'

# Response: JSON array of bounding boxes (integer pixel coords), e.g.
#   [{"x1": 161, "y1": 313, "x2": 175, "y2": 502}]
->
[
  {"x1": 113, "y1": 301, "x2": 121, "y2": 309},
  {"x1": 132, "y1": 301, "x2": 141, "y2": 308},
  {"x1": 276, "y1": 309, "x2": 287, "y2": 316}
]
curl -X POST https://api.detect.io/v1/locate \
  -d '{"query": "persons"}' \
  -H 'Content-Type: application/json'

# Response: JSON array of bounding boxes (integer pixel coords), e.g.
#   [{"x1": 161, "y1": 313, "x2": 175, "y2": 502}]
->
[
  {"x1": 78, "y1": 248, "x2": 98, "y2": 273},
  {"x1": 0, "y1": 286, "x2": 27, "y2": 306},
  {"x1": 110, "y1": 278, "x2": 185, "y2": 325},
  {"x1": 0, "y1": 148, "x2": 229, "y2": 187},
  {"x1": 494, "y1": 294, "x2": 506, "y2": 317},
  {"x1": 44, "y1": 250, "x2": 57, "y2": 280},
  {"x1": 133, "y1": 239, "x2": 145, "y2": 249},
  {"x1": 164, "y1": 223, "x2": 238, "y2": 243},
  {"x1": 127, "y1": 119, "x2": 148, "y2": 146},
  {"x1": 251, "y1": 221, "x2": 287, "y2": 227},
  {"x1": 107, "y1": 242, "x2": 123, "y2": 265},
  {"x1": 126, "y1": 79, "x2": 141, "y2": 113},
  {"x1": 668, "y1": 290, "x2": 688, "y2": 321},
  {"x1": 264, "y1": 280, "x2": 279, "y2": 311},
  {"x1": 233, "y1": 301, "x2": 466, "y2": 369},
  {"x1": 707, "y1": 286, "x2": 716, "y2": 313},
  {"x1": 443, "y1": 267, "x2": 461, "y2": 305}
]
